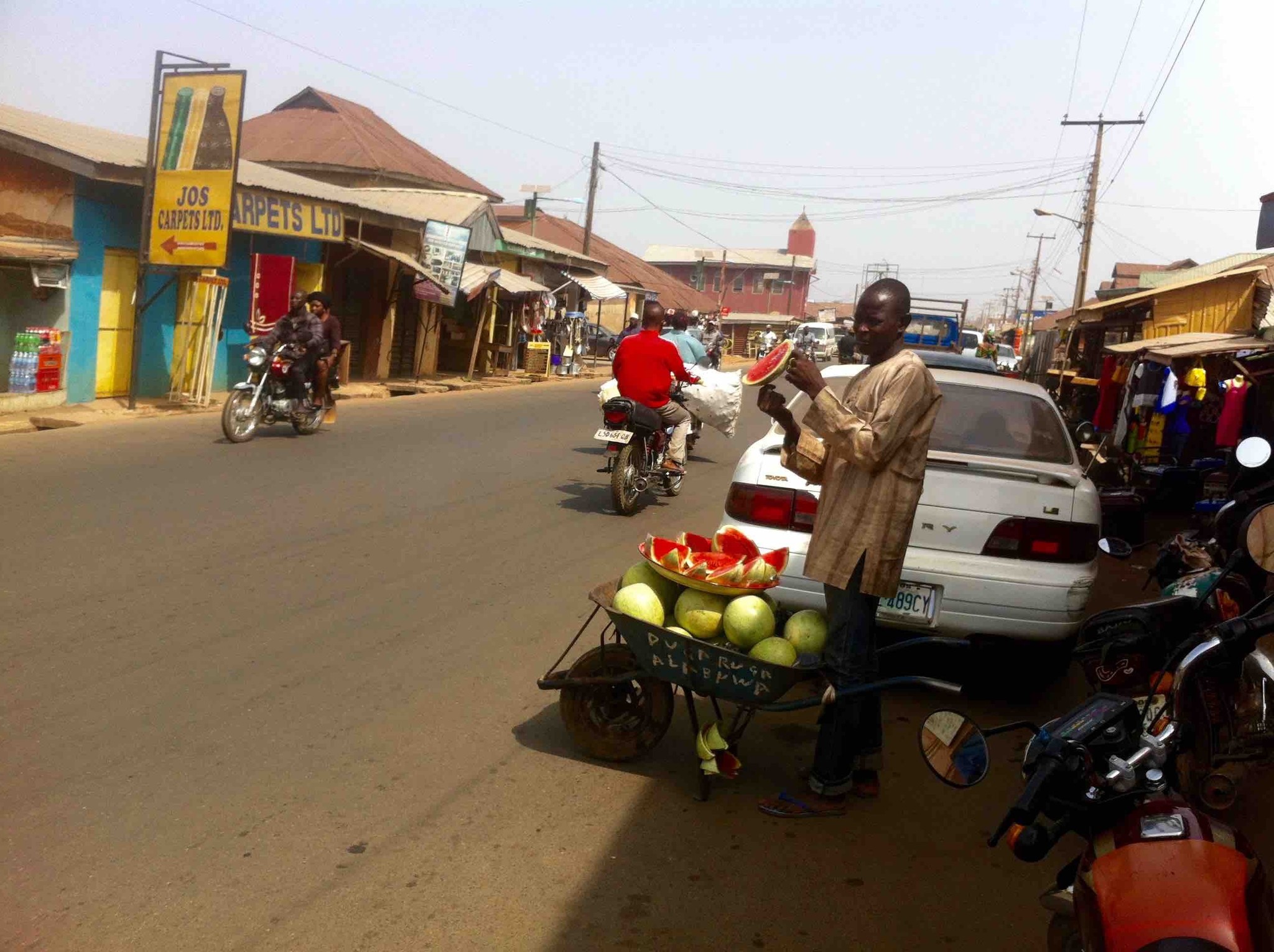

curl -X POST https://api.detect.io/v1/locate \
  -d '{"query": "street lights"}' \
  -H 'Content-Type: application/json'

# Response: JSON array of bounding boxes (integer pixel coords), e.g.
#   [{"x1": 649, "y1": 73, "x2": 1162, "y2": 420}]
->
[
  {"x1": 767, "y1": 279, "x2": 794, "y2": 314},
  {"x1": 531, "y1": 193, "x2": 586, "y2": 235},
  {"x1": 1034, "y1": 209, "x2": 1095, "y2": 316},
  {"x1": 1010, "y1": 272, "x2": 1037, "y2": 355}
]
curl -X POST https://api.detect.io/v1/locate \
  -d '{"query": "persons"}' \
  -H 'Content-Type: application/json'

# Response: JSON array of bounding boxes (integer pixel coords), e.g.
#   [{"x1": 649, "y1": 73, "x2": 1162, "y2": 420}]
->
[
  {"x1": 612, "y1": 299, "x2": 865, "y2": 475},
  {"x1": 259, "y1": 291, "x2": 340, "y2": 415},
  {"x1": 758, "y1": 277, "x2": 943, "y2": 819}
]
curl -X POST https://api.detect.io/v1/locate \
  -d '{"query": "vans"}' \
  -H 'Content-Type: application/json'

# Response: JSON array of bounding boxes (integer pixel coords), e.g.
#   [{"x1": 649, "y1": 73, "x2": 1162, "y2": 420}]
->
[
  {"x1": 957, "y1": 329, "x2": 983, "y2": 359},
  {"x1": 791, "y1": 322, "x2": 834, "y2": 362}
]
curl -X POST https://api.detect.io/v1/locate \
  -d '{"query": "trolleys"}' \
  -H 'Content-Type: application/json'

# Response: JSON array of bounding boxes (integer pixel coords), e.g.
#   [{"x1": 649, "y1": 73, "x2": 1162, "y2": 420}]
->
[{"x1": 537, "y1": 577, "x2": 971, "y2": 801}]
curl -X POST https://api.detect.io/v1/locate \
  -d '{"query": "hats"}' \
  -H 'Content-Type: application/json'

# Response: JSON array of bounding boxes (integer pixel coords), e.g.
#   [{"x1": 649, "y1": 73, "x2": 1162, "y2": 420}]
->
[
  {"x1": 308, "y1": 290, "x2": 327, "y2": 310},
  {"x1": 708, "y1": 321, "x2": 716, "y2": 326},
  {"x1": 766, "y1": 324, "x2": 771, "y2": 328},
  {"x1": 627, "y1": 312, "x2": 639, "y2": 320}
]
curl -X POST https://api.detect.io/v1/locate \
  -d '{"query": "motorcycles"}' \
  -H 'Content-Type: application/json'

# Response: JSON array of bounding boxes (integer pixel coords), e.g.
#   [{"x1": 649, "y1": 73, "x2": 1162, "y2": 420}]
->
[
  {"x1": 920, "y1": 421, "x2": 1274, "y2": 952},
  {"x1": 755, "y1": 338, "x2": 820, "y2": 364},
  {"x1": 221, "y1": 324, "x2": 349, "y2": 442},
  {"x1": 596, "y1": 342, "x2": 722, "y2": 515}
]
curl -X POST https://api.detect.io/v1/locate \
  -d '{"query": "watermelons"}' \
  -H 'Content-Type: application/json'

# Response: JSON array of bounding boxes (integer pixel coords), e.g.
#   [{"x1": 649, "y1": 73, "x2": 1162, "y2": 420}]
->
[
  {"x1": 645, "y1": 526, "x2": 789, "y2": 586},
  {"x1": 741, "y1": 339, "x2": 793, "y2": 385},
  {"x1": 613, "y1": 559, "x2": 828, "y2": 667}
]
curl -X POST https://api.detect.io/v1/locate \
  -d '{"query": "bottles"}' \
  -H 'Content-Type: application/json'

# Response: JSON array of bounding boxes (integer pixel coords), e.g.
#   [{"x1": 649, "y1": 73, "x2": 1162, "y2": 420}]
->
[{"x1": 7, "y1": 326, "x2": 62, "y2": 395}]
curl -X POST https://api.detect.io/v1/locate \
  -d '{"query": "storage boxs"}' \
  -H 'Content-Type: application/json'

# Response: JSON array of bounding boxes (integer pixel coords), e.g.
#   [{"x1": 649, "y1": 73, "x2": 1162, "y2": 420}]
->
[{"x1": 35, "y1": 354, "x2": 62, "y2": 393}]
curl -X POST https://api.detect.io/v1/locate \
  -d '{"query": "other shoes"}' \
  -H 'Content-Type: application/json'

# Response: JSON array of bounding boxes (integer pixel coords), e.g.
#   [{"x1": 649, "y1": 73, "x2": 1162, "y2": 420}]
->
[
  {"x1": 311, "y1": 401, "x2": 323, "y2": 410},
  {"x1": 291, "y1": 407, "x2": 308, "y2": 420}
]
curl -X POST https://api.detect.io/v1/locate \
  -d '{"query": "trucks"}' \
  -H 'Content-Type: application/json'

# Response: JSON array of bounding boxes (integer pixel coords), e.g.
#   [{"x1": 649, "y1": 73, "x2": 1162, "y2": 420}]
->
[{"x1": 906, "y1": 297, "x2": 968, "y2": 357}]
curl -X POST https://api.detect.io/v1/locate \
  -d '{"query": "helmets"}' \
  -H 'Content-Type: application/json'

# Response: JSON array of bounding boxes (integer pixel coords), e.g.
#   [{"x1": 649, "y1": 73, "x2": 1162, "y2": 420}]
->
[{"x1": 672, "y1": 312, "x2": 689, "y2": 328}]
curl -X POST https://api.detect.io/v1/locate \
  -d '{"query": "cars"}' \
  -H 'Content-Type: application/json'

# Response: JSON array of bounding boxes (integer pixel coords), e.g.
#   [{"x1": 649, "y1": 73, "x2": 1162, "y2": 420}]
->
[
  {"x1": 714, "y1": 350, "x2": 1102, "y2": 683},
  {"x1": 994, "y1": 342, "x2": 1023, "y2": 370},
  {"x1": 586, "y1": 324, "x2": 702, "y2": 361},
  {"x1": 835, "y1": 335, "x2": 845, "y2": 352}
]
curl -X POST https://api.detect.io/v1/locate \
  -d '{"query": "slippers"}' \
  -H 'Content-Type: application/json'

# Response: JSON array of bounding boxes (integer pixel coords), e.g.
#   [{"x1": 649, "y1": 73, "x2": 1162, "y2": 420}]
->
[
  {"x1": 757, "y1": 788, "x2": 848, "y2": 818},
  {"x1": 658, "y1": 461, "x2": 685, "y2": 474},
  {"x1": 794, "y1": 765, "x2": 877, "y2": 796}
]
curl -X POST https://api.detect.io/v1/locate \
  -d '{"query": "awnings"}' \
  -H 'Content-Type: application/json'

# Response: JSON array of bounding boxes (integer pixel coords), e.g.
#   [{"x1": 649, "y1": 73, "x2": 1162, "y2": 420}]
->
[
  {"x1": 346, "y1": 235, "x2": 552, "y2": 301},
  {"x1": 550, "y1": 264, "x2": 627, "y2": 301}
]
